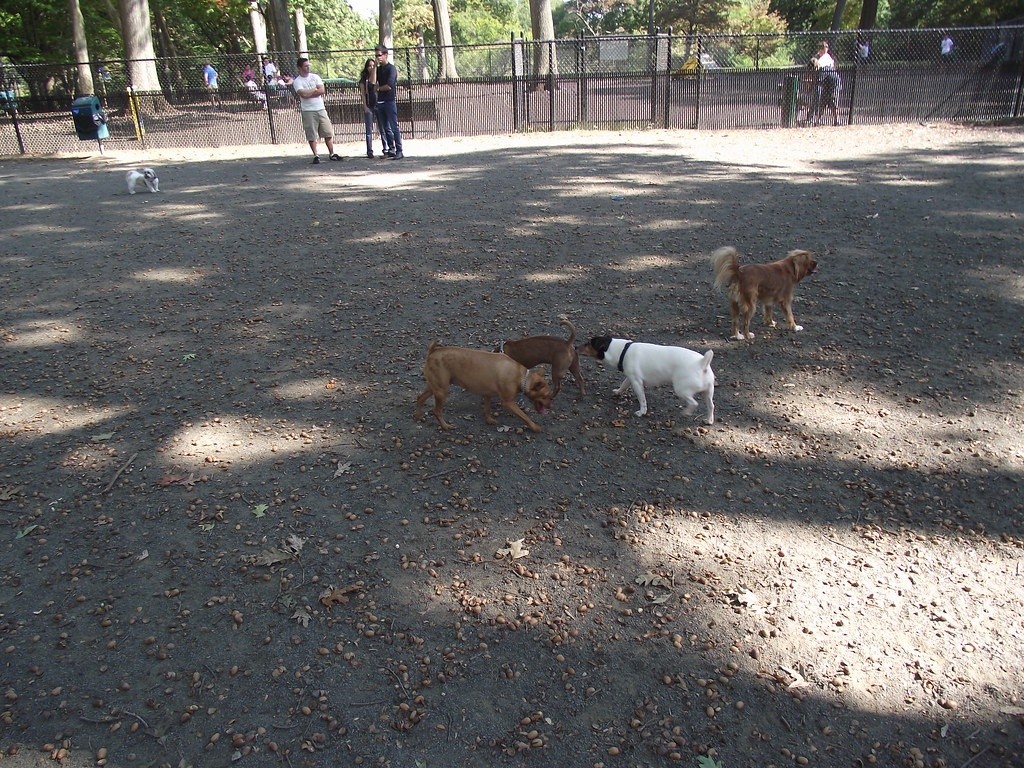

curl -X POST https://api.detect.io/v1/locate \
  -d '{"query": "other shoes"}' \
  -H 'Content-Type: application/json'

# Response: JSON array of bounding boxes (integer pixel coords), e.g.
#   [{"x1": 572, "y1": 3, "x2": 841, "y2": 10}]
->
[
  {"x1": 329, "y1": 154, "x2": 343, "y2": 161},
  {"x1": 313, "y1": 156, "x2": 321, "y2": 164},
  {"x1": 368, "y1": 154, "x2": 374, "y2": 159},
  {"x1": 387, "y1": 153, "x2": 396, "y2": 160},
  {"x1": 393, "y1": 152, "x2": 404, "y2": 160},
  {"x1": 380, "y1": 153, "x2": 389, "y2": 159}
]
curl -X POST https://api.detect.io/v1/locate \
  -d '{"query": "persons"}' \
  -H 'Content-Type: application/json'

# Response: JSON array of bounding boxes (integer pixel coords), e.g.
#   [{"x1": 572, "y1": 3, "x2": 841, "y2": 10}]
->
[
  {"x1": 293, "y1": 57, "x2": 343, "y2": 165},
  {"x1": 359, "y1": 59, "x2": 389, "y2": 158},
  {"x1": 367, "y1": 44, "x2": 404, "y2": 160},
  {"x1": 807, "y1": 39, "x2": 871, "y2": 126},
  {"x1": 0, "y1": 87, "x2": 21, "y2": 118},
  {"x1": 941, "y1": 32, "x2": 954, "y2": 64},
  {"x1": 170, "y1": 45, "x2": 293, "y2": 109}
]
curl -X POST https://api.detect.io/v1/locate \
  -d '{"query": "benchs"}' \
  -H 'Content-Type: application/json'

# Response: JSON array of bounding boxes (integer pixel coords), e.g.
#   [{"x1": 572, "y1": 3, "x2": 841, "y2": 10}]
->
[{"x1": 325, "y1": 99, "x2": 440, "y2": 134}]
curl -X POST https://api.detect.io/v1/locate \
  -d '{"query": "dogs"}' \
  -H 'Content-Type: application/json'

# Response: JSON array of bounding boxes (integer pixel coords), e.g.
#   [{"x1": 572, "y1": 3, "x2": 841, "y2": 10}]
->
[
  {"x1": 414, "y1": 321, "x2": 586, "y2": 430},
  {"x1": 574, "y1": 332, "x2": 717, "y2": 427},
  {"x1": 711, "y1": 242, "x2": 819, "y2": 342},
  {"x1": 124, "y1": 168, "x2": 160, "y2": 195}
]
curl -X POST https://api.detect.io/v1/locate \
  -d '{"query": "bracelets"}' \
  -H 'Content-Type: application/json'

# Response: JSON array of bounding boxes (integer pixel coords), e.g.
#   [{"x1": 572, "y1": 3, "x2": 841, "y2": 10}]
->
[{"x1": 377, "y1": 87, "x2": 380, "y2": 92}]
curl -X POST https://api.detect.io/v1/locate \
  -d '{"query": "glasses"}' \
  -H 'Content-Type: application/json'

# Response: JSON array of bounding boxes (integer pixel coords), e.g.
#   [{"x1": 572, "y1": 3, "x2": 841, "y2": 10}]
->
[{"x1": 375, "y1": 54, "x2": 382, "y2": 57}]
